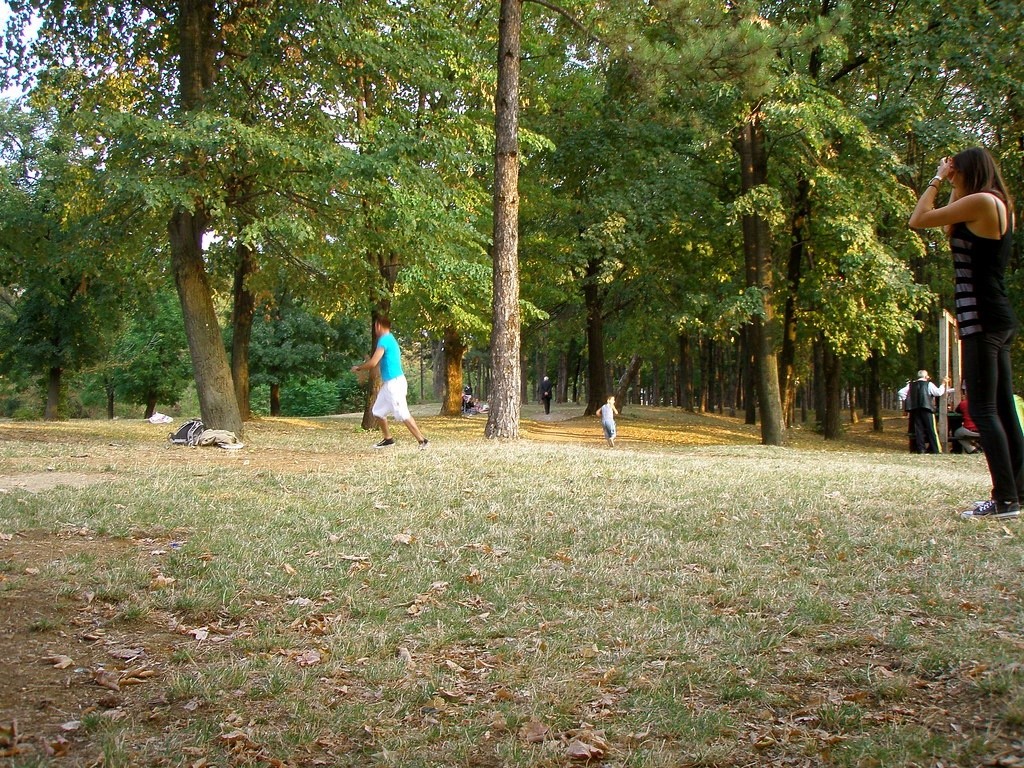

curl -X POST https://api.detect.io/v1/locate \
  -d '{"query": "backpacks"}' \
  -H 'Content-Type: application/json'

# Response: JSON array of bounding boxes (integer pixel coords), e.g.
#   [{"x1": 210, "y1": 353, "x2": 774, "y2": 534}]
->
[{"x1": 170, "y1": 420, "x2": 203, "y2": 444}]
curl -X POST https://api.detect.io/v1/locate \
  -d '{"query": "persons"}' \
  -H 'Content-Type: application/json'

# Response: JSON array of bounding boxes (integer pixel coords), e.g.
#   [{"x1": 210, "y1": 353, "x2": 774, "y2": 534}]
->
[
  {"x1": 954, "y1": 391, "x2": 982, "y2": 453},
  {"x1": 539, "y1": 376, "x2": 553, "y2": 414},
  {"x1": 910, "y1": 148, "x2": 1024, "y2": 519},
  {"x1": 595, "y1": 395, "x2": 619, "y2": 447},
  {"x1": 898, "y1": 370, "x2": 945, "y2": 453},
  {"x1": 350, "y1": 313, "x2": 428, "y2": 447}
]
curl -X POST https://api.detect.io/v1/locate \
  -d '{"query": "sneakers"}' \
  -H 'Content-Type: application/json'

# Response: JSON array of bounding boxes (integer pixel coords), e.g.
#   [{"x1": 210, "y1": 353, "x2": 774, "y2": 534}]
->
[
  {"x1": 961, "y1": 498, "x2": 1023, "y2": 521},
  {"x1": 417, "y1": 440, "x2": 430, "y2": 449},
  {"x1": 374, "y1": 438, "x2": 395, "y2": 448}
]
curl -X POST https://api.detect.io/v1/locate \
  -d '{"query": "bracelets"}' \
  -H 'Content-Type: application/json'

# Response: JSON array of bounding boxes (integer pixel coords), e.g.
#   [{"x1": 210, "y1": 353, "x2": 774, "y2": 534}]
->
[{"x1": 929, "y1": 176, "x2": 943, "y2": 185}]
